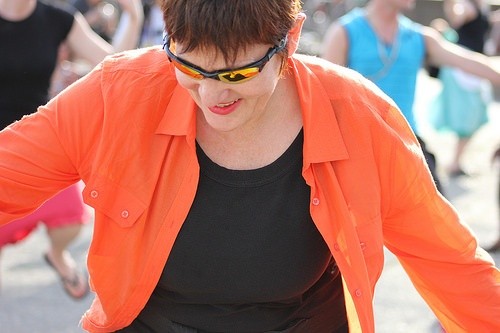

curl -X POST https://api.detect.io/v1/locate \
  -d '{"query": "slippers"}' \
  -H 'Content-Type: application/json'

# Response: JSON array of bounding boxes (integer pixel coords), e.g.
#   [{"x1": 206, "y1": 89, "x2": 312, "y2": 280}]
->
[{"x1": 44, "y1": 253, "x2": 87, "y2": 299}]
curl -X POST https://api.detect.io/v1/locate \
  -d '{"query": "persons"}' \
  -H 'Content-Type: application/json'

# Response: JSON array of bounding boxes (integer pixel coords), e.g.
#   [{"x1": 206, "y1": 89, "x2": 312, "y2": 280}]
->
[{"x1": 0, "y1": 0, "x2": 500, "y2": 333}]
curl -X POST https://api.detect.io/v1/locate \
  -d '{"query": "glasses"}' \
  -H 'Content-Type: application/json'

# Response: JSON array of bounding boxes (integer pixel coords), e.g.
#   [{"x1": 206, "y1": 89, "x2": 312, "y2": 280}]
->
[{"x1": 162, "y1": 29, "x2": 287, "y2": 83}]
